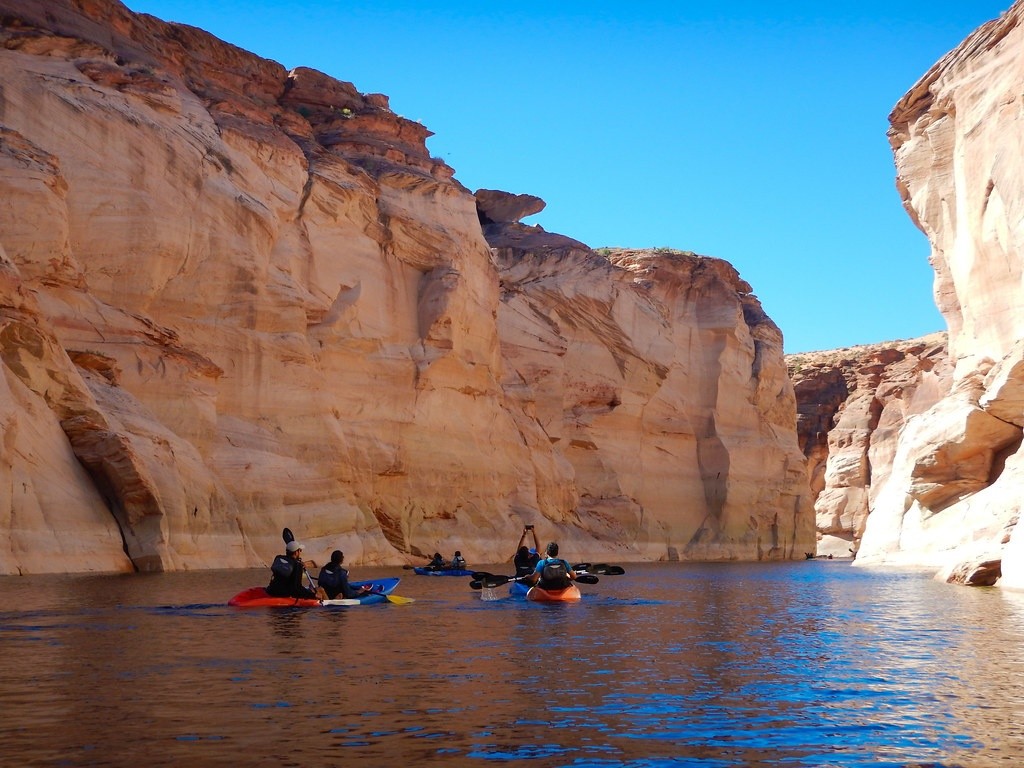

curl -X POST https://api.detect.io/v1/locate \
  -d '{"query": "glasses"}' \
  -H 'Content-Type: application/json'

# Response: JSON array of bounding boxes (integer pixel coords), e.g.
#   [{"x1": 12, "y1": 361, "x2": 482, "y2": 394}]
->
[
  {"x1": 343, "y1": 556, "x2": 344, "y2": 558},
  {"x1": 298, "y1": 549, "x2": 302, "y2": 552}
]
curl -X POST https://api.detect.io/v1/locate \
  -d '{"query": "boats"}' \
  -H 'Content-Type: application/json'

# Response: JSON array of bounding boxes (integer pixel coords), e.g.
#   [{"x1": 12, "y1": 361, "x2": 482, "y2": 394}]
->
[
  {"x1": 227, "y1": 576, "x2": 402, "y2": 608},
  {"x1": 508, "y1": 580, "x2": 532, "y2": 597},
  {"x1": 526, "y1": 578, "x2": 582, "y2": 601},
  {"x1": 413, "y1": 565, "x2": 476, "y2": 577}
]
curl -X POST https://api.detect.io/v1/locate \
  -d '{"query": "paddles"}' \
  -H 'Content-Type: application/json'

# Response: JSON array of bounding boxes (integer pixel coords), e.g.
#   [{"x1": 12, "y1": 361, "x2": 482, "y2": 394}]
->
[
  {"x1": 470, "y1": 563, "x2": 626, "y2": 589},
  {"x1": 283, "y1": 527, "x2": 318, "y2": 594},
  {"x1": 402, "y1": 565, "x2": 432, "y2": 571},
  {"x1": 302, "y1": 572, "x2": 414, "y2": 605},
  {"x1": 481, "y1": 561, "x2": 611, "y2": 589}
]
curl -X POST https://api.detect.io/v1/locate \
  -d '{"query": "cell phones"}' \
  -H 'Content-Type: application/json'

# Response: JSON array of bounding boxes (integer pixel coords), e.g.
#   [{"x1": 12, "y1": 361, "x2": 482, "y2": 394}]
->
[{"x1": 525, "y1": 524, "x2": 534, "y2": 530}]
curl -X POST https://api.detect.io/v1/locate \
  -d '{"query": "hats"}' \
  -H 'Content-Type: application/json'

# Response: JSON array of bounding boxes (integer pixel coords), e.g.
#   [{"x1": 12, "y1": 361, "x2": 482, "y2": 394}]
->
[
  {"x1": 454, "y1": 551, "x2": 461, "y2": 556},
  {"x1": 287, "y1": 541, "x2": 305, "y2": 552},
  {"x1": 331, "y1": 550, "x2": 343, "y2": 564},
  {"x1": 434, "y1": 553, "x2": 440, "y2": 557}
]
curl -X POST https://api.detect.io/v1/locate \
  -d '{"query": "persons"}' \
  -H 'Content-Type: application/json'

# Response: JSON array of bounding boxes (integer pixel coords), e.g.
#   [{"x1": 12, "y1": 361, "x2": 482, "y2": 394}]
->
[
  {"x1": 267, "y1": 541, "x2": 343, "y2": 600},
  {"x1": 525, "y1": 542, "x2": 577, "y2": 589},
  {"x1": 806, "y1": 553, "x2": 813, "y2": 558},
  {"x1": 451, "y1": 550, "x2": 466, "y2": 570},
  {"x1": 513, "y1": 527, "x2": 541, "y2": 583},
  {"x1": 427, "y1": 553, "x2": 446, "y2": 571},
  {"x1": 318, "y1": 550, "x2": 384, "y2": 599}
]
